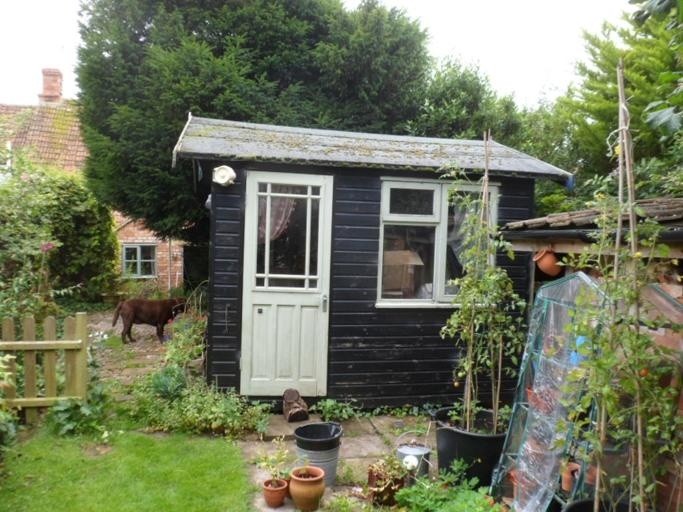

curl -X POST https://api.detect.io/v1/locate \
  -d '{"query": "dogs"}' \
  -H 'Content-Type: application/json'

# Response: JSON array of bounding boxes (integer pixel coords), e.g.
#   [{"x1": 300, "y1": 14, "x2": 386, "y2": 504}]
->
[{"x1": 112, "y1": 296, "x2": 190, "y2": 344}]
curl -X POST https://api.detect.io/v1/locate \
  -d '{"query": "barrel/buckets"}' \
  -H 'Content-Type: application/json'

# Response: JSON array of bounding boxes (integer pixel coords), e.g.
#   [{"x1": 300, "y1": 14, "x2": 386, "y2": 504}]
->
[{"x1": 393, "y1": 429, "x2": 431, "y2": 488}]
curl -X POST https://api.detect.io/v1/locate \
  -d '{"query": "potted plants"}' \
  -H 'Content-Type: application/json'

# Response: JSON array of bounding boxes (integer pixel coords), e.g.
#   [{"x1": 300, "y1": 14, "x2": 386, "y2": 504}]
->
[
  {"x1": 427, "y1": 127, "x2": 530, "y2": 491},
  {"x1": 360, "y1": 451, "x2": 408, "y2": 507},
  {"x1": 246, "y1": 411, "x2": 327, "y2": 512}
]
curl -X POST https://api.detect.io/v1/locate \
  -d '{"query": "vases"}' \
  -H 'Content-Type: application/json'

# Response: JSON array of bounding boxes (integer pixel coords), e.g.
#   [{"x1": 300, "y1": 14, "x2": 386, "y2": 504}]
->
[
  {"x1": 533, "y1": 248, "x2": 560, "y2": 279},
  {"x1": 512, "y1": 334, "x2": 603, "y2": 503}
]
